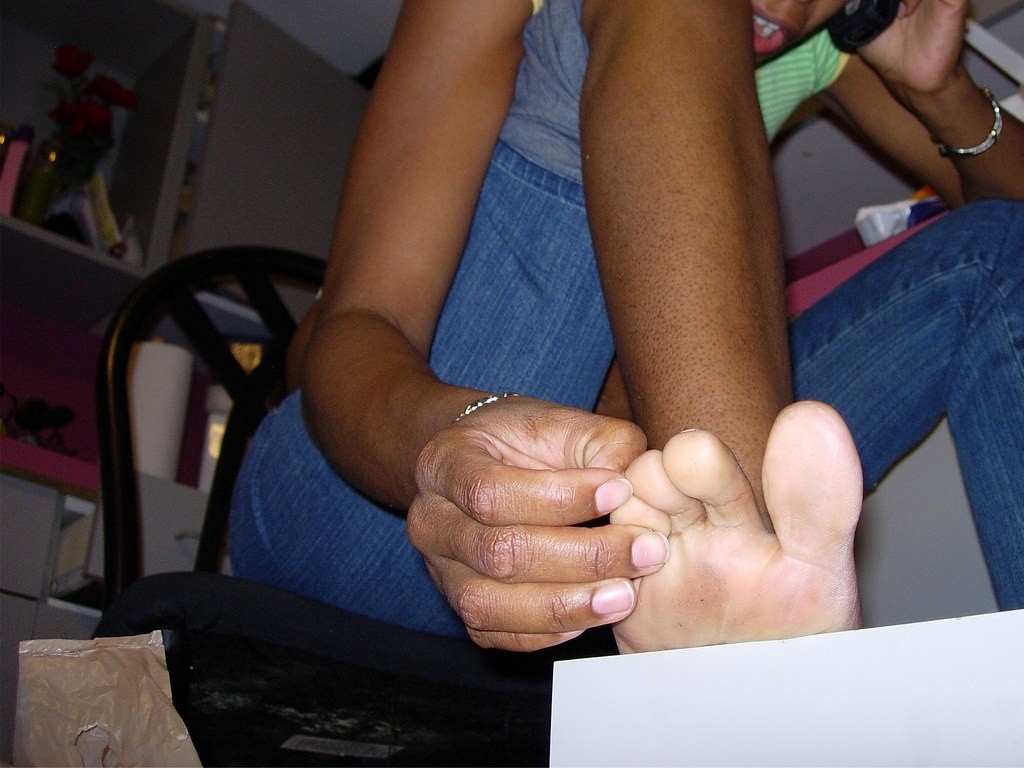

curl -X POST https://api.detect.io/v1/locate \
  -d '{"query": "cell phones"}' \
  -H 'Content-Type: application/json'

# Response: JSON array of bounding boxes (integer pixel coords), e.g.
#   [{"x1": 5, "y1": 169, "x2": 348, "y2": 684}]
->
[{"x1": 828, "y1": 0, "x2": 901, "y2": 53}]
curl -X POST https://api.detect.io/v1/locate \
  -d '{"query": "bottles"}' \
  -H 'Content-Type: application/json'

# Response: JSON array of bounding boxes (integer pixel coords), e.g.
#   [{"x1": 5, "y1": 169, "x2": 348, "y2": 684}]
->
[
  {"x1": 12, "y1": 141, "x2": 53, "y2": 222},
  {"x1": 0, "y1": 124, "x2": 33, "y2": 215}
]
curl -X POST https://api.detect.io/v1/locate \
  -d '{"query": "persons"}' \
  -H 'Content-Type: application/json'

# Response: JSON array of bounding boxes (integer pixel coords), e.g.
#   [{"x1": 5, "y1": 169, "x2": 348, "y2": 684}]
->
[{"x1": 222, "y1": 0, "x2": 1023, "y2": 654}]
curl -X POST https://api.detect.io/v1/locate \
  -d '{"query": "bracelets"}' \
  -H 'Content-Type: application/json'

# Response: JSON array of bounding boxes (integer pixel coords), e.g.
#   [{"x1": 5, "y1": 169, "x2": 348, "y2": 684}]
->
[
  {"x1": 928, "y1": 87, "x2": 1003, "y2": 158},
  {"x1": 450, "y1": 393, "x2": 519, "y2": 426}
]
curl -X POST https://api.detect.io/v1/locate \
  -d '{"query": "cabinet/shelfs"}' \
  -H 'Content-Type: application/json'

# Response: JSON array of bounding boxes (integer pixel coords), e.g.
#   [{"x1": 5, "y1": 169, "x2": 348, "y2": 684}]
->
[
  {"x1": 0, "y1": 0, "x2": 368, "y2": 346},
  {"x1": 0, "y1": 474, "x2": 103, "y2": 768}
]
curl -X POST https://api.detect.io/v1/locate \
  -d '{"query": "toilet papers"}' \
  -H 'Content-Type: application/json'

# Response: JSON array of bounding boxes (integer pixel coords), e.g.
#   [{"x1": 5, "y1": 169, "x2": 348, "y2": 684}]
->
[{"x1": 126, "y1": 341, "x2": 196, "y2": 484}]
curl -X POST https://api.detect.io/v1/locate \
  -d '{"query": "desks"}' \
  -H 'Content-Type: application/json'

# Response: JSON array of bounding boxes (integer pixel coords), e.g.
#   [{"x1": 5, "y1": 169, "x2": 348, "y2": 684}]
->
[{"x1": 550, "y1": 606, "x2": 1022, "y2": 768}]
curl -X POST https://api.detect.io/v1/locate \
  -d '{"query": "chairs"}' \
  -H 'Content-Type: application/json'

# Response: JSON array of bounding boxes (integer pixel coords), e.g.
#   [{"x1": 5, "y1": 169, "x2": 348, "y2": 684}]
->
[{"x1": 88, "y1": 245, "x2": 619, "y2": 768}]
